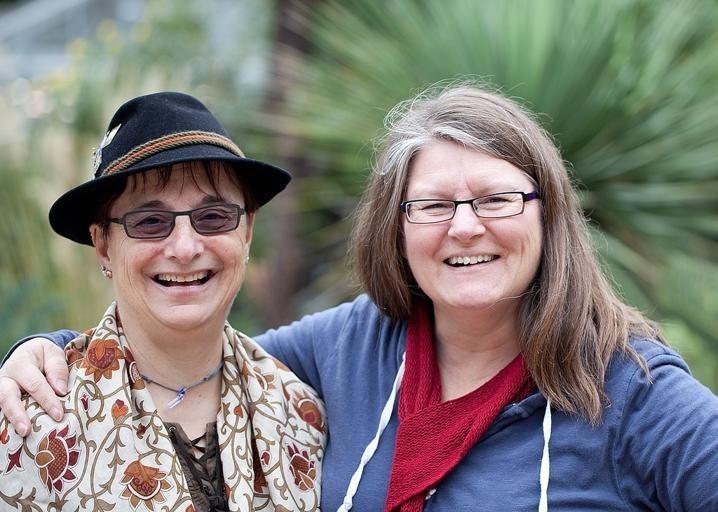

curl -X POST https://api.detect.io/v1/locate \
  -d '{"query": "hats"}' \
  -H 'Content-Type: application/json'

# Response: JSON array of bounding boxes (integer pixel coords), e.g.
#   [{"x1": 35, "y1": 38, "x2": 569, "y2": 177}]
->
[{"x1": 48, "y1": 91, "x2": 292, "y2": 247}]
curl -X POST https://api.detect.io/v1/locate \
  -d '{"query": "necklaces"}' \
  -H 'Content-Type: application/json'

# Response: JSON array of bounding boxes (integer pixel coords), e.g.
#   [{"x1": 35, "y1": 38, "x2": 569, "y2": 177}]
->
[{"x1": 133, "y1": 362, "x2": 223, "y2": 408}]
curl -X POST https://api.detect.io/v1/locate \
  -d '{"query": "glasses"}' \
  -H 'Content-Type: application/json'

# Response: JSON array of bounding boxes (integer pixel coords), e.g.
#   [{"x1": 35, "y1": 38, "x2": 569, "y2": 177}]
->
[
  {"x1": 110, "y1": 202, "x2": 247, "y2": 239},
  {"x1": 398, "y1": 191, "x2": 539, "y2": 223}
]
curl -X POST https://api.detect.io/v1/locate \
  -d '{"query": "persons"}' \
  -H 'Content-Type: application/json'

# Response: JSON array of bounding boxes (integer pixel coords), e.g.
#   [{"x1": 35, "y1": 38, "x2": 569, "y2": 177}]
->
[
  {"x1": 0, "y1": 78, "x2": 718, "y2": 512},
  {"x1": 0, "y1": 91, "x2": 328, "y2": 512}
]
